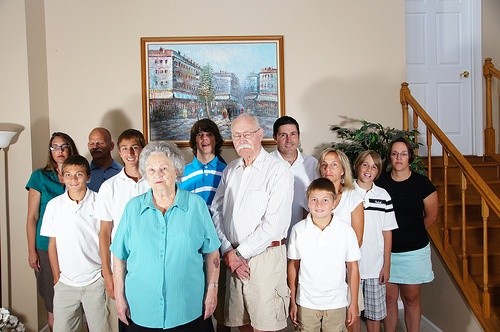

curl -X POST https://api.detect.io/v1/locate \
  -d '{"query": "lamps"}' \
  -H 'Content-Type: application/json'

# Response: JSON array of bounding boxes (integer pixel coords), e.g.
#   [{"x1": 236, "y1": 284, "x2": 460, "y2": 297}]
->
[{"x1": 0, "y1": 130, "x2": 18, "y2": 310}]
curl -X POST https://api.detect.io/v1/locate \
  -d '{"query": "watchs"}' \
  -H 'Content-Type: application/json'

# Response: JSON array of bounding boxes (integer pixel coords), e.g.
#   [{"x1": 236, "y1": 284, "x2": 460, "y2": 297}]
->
[{"x1": 236, "y1": 250, "x2": 243, "y2": 258}]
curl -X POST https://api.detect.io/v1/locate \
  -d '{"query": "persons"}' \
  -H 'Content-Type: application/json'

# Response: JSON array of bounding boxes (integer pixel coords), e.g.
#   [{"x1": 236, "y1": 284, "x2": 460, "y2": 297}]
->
[
  {"x1": 300, "y1": 149, "x2": 366, "y2": 332},
  {"x1": 211, "y1": 113, "x2": 291, "y2": 332},
  {"x1": 174, "y1": 118, "x2": 227, "y2": 213},
  {"x1": 86, "y1": 128, "x2": 123, "y2": 193},
  {"x1": 25, "y1": 132, "x2": 79, "y2": 332},
  {"x1": 110, "y1": 141, "x2": 222, "y2": 332},
  {"x1": 374, "y1": 137, "x2": 438, "y2": 332},
  {"x1": 351, "y1": 151, "x2": 399, "y2": 332},
  {"x1": 271, "y1": 116, "x2": 320, "y2": 230},
  {"x1": 288, "y1": 178, "x2": 361, "y2": 332},
  {"x1": 40, "y1": 155, "x2": 112, "y2": 332},
  {"x1": 93, "y1": 129, "x2": 149, "y2": 332}
]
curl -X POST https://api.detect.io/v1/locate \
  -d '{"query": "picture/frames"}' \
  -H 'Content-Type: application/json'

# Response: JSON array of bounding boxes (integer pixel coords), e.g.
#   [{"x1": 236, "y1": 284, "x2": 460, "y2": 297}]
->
[{"x1": 138, "y1": 34, "x2": 287, "y2": 148}]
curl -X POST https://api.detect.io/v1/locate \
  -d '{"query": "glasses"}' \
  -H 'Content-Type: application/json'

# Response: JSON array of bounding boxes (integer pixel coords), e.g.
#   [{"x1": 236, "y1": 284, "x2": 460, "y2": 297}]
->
[
  {"x1": 232, "y1": 128, "x2": 260, "y2": 141},
  {"x1": 49, "y1": 144, "x2": 72, "y2": 150},
  {"x1": 390, "y1": 150, "x2": 409, "y2": 158}
]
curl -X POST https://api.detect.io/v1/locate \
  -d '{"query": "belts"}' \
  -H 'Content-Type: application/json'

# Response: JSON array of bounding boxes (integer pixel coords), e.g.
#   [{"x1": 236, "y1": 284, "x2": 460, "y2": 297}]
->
[{"x1": 232, "y1": 239, "x2": 288, "y2": 250}]
